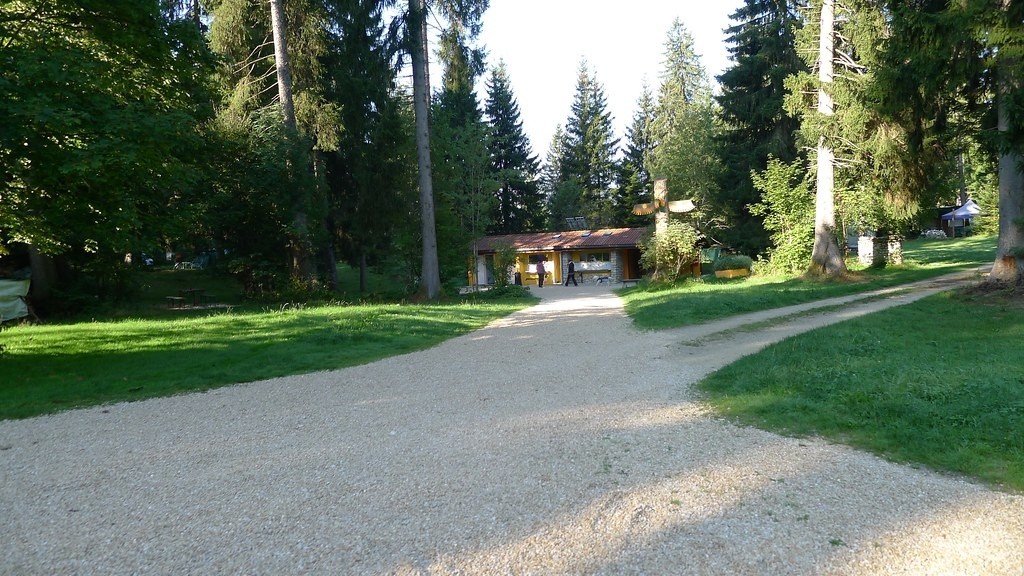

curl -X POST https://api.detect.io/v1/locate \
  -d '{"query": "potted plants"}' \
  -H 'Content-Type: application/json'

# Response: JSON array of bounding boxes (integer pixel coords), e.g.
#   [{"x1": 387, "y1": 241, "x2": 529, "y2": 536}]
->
[{"x1": 713, "y1": 253, "x2": 754, "y2": 279}]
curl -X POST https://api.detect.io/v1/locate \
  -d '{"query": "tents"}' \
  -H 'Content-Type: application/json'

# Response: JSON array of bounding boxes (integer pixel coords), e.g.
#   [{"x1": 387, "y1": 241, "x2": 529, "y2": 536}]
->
[{"x1": 941, "y1": 199, "x2": 989, "y2": 238}]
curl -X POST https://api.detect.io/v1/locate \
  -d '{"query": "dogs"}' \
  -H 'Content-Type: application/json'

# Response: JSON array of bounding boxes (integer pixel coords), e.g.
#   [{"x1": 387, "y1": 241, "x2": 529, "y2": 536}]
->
[{"x1": 592, "y1": 276, "x2": 615, "y2": 286}]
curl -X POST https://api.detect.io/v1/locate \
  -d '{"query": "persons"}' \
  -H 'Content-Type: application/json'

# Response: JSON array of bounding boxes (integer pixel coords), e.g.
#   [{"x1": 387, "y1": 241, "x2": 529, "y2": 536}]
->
[
  {"x1": 536, "y1": 259, "x2": 547, "y2": 289},
  {"x1": 564, "y1": 258, "x2": 578, "y2": 287}
]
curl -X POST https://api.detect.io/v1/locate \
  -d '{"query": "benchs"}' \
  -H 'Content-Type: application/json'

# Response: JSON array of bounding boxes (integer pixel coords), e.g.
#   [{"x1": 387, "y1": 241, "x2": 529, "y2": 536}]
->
[
  {"x1": 199, "y1": 293, "x2": 217, "y2": 307},
  {"x1": 166, "y1": 295, "x2": 187, "y2": 309}
]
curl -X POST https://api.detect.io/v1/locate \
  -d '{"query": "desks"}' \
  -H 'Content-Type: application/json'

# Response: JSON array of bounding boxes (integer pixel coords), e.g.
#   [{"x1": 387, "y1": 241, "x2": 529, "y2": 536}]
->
[{"x1": 177, "y1": 288, "x2": 205, "y2": 308}]
